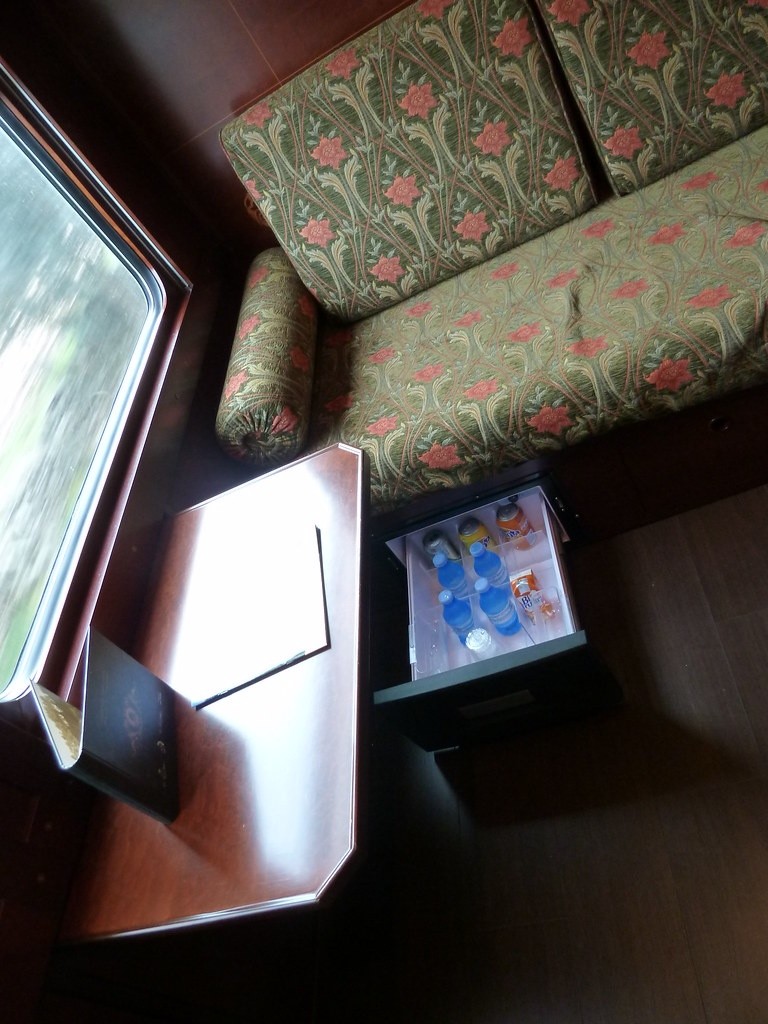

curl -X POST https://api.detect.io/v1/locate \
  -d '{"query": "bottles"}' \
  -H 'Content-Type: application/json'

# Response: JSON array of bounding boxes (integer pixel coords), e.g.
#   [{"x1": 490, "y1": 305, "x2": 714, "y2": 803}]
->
[
  {"x1": 439, "y1": 590, "x2": 496, "y2": 659},
  {"x1": 470, "y1": 543, "x2": 509, "y2": 586},
  {"x1": 432, "y1": 552, "x2": 470, "y2": 597},
  {"x1": 472, "y1": 579, "x2": 522, "y2": 636}
]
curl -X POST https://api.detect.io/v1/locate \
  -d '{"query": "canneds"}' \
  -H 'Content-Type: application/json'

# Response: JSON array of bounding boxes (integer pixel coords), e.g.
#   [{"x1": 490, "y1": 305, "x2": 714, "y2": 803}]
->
[
  {"x1": 458, "y1": 518, "x2": 500, "y2": 555},
  {"x1": 421, "y1": 530, "x2": 461, "y2": 564},
  {"x1": 496, "y1": 503, "x2": 538, "y2": 551}
]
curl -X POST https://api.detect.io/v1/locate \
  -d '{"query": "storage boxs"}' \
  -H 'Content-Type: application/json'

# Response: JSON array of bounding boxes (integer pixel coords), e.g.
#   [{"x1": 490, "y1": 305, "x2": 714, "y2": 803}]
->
[{"x1": 365, "y1": 477, "x2": 608, "y2": 755}]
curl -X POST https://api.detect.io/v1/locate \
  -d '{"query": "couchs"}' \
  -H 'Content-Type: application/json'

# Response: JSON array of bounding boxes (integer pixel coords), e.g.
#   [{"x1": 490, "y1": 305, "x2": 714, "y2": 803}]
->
[{"x1": 214, "y1": 0, "x2": 768, "y2": 525}]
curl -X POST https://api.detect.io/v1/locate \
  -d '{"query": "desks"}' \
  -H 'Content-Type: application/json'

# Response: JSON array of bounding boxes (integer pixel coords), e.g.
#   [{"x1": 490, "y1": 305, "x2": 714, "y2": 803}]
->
[{"x1": 56, "y1": 444, "x2": 370, "y2": 952}]
